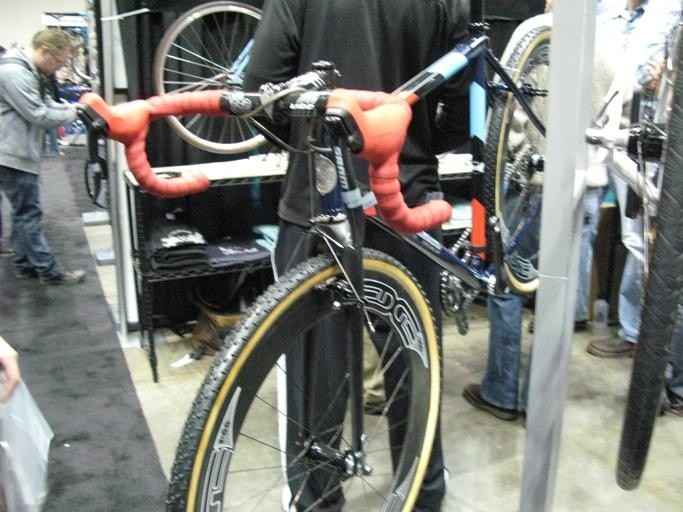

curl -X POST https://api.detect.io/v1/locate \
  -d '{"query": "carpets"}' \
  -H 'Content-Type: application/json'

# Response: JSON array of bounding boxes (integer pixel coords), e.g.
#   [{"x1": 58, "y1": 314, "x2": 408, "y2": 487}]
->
[{"x1": 1, "y1": 130, "x2": 172, "y2": 512}]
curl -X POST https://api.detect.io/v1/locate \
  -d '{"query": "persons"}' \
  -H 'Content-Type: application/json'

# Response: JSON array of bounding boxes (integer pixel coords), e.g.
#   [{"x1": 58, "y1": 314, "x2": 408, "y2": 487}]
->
[
  {"x1": 0, "y1": 334, "x2": 23, "y2": 404},
  {"x1": 246, "y1": 0, "x2": 472, "y2": 511},
  {"x1": 38, "y1": 69, "x2": 66, "y2": 156},
  {"x1": 0, "y1": 25, "x2": 87, "y2": 288}
]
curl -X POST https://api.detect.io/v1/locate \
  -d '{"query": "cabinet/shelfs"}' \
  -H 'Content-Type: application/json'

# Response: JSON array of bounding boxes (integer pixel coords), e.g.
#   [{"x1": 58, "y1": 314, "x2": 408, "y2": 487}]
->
[{"x1": 124, "y1": 135, "x2": 486, "y2": 384}]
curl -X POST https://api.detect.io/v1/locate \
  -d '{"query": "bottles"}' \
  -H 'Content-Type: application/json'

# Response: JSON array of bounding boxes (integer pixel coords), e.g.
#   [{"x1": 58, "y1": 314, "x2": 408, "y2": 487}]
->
[{"x1": 593, "y1": 294, "x2": 609, "y2": 329}]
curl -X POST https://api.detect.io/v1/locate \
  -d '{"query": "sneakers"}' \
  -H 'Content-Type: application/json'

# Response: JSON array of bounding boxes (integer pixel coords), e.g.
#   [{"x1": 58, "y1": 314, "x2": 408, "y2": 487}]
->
[
  {"x1": 40, "y1": 267, "x2": 85, "y2": 286},
  {"x1": 463, "y1": 383, "x2": 519, "y2": 422},
  {"x1": 587, "y1": 334, "x2": 640, "y2": 359},
  {"x1": 13, "y1": 263, "x2": 37, "y2": 281}
]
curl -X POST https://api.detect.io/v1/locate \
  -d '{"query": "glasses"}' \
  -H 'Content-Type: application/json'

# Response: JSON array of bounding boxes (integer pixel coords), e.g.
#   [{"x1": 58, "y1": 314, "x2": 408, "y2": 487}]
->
[{"x1": 50, "y1": 53, "x2": 65, "y2": 72}]
